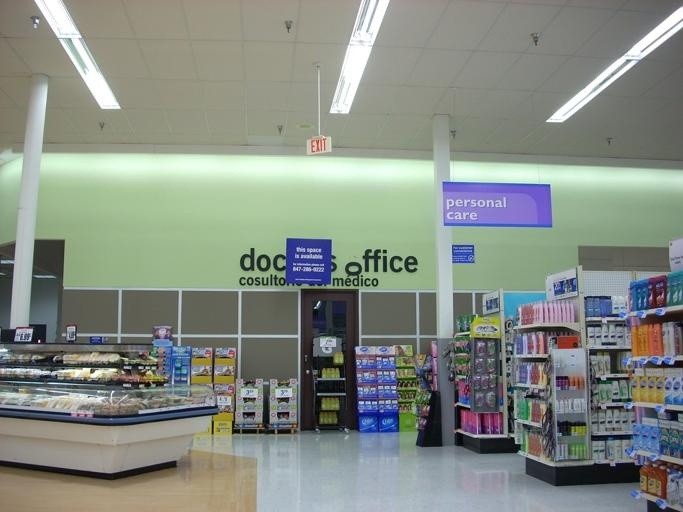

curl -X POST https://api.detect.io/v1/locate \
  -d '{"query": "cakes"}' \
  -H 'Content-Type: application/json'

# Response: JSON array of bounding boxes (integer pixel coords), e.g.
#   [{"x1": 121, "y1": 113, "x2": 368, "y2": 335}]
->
[
  {"x1": 63, "y1": 352, "x2": 121, "y2": 366},
  {"x1": 46, "y1": 397, "x2": 140, "y2": 416},
  {"x1": 0, "y1": 348, "x2": 46, "y2": 362},
  {"x1": 0, "y1": 368, "x2": 50, "y2": 381},
  {"x1": 1, "y1": 393, "x2": 45, "y2": 408},
  {"x1": 52, "y1": 368, "x2": 116, "y2": 382}
]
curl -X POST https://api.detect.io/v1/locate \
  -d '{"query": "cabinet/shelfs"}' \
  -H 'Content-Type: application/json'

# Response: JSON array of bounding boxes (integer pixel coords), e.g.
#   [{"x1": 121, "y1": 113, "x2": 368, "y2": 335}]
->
[
  {"x1": 0, "y1": 380, "x2": 223, "y2": 483},
  {"x1": 1, "y1": 340, "x2": 169, "y2": 389}
]
